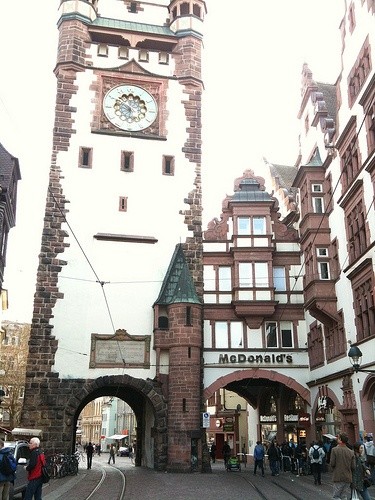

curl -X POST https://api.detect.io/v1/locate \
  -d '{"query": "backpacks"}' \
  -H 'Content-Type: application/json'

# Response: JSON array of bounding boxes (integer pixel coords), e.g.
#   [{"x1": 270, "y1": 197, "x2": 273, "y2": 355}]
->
[
  {"x1": 0, "y1": 453, "x2": 16, "y2": 477},
  {"x1": 312, "y1": 446, "x2": 320, "y2": 459}
]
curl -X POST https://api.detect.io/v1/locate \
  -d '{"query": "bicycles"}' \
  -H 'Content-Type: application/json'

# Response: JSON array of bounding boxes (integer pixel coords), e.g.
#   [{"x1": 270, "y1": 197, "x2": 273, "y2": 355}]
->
[{"x1": 44, "y1": 452, "x2": 80, "y2": 479}]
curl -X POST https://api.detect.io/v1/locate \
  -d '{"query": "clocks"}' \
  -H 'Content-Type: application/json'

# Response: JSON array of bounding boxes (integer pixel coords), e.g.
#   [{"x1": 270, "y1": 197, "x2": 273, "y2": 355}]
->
[
  {"x1": 103, "y1": 84, "x2": 158, "y2": 131},
  {"x1": 215, "y1": 419, "x2": 221, "y2": 428}
]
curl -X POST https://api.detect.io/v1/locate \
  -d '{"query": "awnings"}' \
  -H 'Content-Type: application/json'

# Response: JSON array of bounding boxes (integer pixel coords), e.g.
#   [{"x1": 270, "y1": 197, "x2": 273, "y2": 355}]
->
[
  {"x1": 12, "y1": 428, "x2": 43, "y2": 439},
  {"x1": 107, "y1": 434, "x2": 128, "y2": 440}
]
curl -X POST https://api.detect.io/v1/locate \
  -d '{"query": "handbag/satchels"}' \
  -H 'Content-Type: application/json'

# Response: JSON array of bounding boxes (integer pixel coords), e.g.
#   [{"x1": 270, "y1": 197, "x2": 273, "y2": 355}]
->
[
  {"x1": 352, "y1": 489, "x2": 360, "y2": 500},
  {"x1": 38, "y1": 448, "x2": 50, "y2": 483},
  {"x1": 363, "y1": 475, "x2": 372, "y2": 488},
  {"x1": 366, "y1": 455, "x2": 375, "y2": 464}
]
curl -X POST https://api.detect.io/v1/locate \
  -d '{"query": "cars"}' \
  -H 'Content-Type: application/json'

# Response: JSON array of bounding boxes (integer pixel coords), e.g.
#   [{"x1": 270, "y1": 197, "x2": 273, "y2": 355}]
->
[
  {"x1": 117, "y1": 446, "x2": 129, "y2": 457},
  {"x1": 0, "y1": 439, "x2": 31, "y2": 500}
]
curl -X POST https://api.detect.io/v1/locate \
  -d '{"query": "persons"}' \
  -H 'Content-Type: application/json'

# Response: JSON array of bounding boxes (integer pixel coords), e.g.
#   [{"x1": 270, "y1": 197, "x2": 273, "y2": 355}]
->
[
  {"x1": 253, "y1": 440, "x2": 265, "y2": 478},
  {"x1": 23, "y1": 437, "x2": 46, "y2": 500},
  {"x1": 330, "y1": 432, "x2": 354, "y2": 500},
  {"x1": 350, "y1": 442, "x2": 371, "y2": 500},
  {"x1": 268, "y1": 435, "x2": 375, "y2": 476},
  {"x1": 221, "y1": 440, "x2": 232, "y2": 470},
  {"x1": 85, "y1": 442, "x2": 95, "y2": 470},
  {"x1": 211, "y1": 442, "x2": 219, "y2": 464},
  {"x1": 0, "y1": 439, "x2": 16, "y2": 500},
  {"x1": 309, "y1": 440, "x2": 326, "y2": 485},
  {"x1": 107, "y1": 444, "x2": 117, "y2": 464}
]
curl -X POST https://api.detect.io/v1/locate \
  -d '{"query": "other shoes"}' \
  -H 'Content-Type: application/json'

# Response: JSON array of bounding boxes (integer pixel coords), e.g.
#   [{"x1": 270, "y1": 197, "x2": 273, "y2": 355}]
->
[
  {"x1": 314, "y1": 480, "x2": 321, "y2": 485},
  {"x1": 272, "y1": 473, "x2": 280, "y2": 476},
  {"x1": 87, "y1": 467, "x2": 91, "y2": 470}
]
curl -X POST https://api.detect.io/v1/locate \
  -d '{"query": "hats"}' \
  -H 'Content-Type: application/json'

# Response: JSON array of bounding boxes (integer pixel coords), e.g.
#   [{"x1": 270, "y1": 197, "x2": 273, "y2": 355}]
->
[
  {"x1": 365, "y1": 433, "x2": 368, "y2": 436},
  {"x1": 368, "y1": 433, "x2": 373, "y2": 437}
]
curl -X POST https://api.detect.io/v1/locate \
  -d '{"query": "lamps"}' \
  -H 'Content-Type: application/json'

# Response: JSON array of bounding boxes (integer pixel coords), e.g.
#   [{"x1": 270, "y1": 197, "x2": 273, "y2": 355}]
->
[{"x1": 348, "y1": 345, "x2": 375, "y2": 374}]
